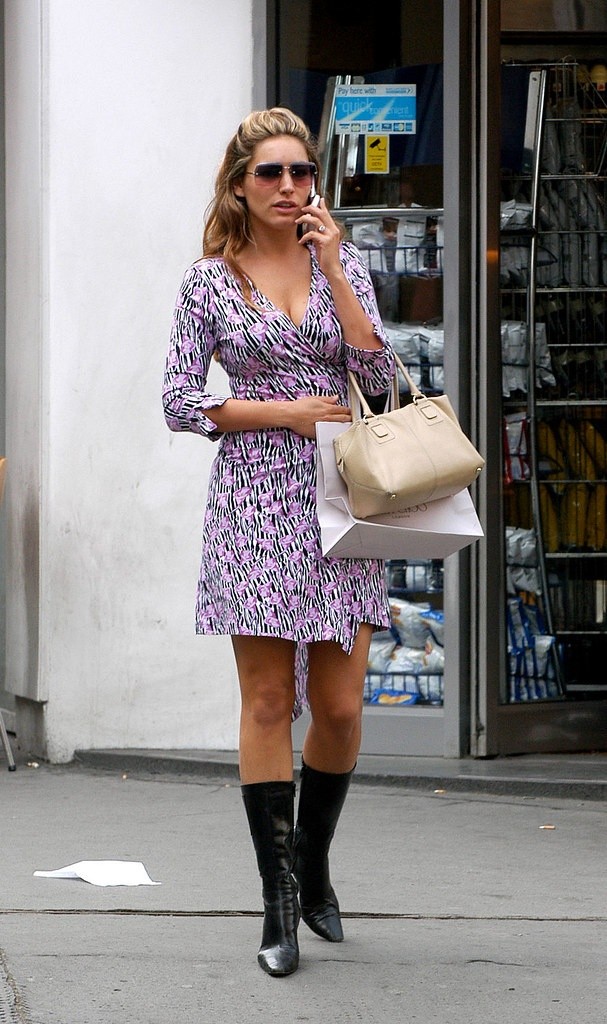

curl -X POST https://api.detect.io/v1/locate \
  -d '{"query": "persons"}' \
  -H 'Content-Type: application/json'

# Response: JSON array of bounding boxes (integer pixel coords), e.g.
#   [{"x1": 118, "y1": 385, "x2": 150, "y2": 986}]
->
[{"x1": 162, "y1": 106, "x2": 394, "y2": 977}]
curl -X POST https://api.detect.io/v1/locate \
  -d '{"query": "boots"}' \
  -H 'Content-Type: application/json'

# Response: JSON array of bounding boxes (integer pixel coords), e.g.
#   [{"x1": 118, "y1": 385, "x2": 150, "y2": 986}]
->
[
  {"x1": 238, "y1": 778, "x2": 302, "y2": 979},
  {"x1": 296, "y1": 755, "x2": 358, "y2": 942}
]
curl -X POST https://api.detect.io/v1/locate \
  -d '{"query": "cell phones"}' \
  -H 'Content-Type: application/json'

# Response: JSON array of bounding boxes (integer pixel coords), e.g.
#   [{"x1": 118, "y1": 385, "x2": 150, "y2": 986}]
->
[{"x1": 301, "y1": 174, "x2": 321, "y2": 236}]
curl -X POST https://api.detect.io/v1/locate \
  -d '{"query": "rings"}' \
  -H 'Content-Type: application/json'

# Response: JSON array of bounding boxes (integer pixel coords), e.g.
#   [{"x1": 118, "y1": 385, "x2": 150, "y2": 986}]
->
[{"x1": 319, "y1": 224, "x2": 327, "y2": 232}]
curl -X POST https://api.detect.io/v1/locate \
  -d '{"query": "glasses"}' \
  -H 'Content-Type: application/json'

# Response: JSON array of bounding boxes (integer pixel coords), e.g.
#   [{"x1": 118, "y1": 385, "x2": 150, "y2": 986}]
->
[{"x1": 244, "y1": 161, "x2": 319, "y2": 189}]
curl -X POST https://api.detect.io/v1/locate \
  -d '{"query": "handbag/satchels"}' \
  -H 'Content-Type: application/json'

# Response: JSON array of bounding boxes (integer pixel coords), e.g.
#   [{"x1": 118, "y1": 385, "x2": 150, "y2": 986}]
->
[{"x1": 314, "y1": 350, "x2": 486, "y2": 559}]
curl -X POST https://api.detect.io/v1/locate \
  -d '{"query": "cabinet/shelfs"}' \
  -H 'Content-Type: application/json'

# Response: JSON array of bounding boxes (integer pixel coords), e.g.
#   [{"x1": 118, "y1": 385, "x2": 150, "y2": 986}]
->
[
  {"x1": 494, "y1": 56, "x2": 607, "y2": 700},
  {"x1": 327, "y1": 203, "x2": 446, "y2": 708}
]
